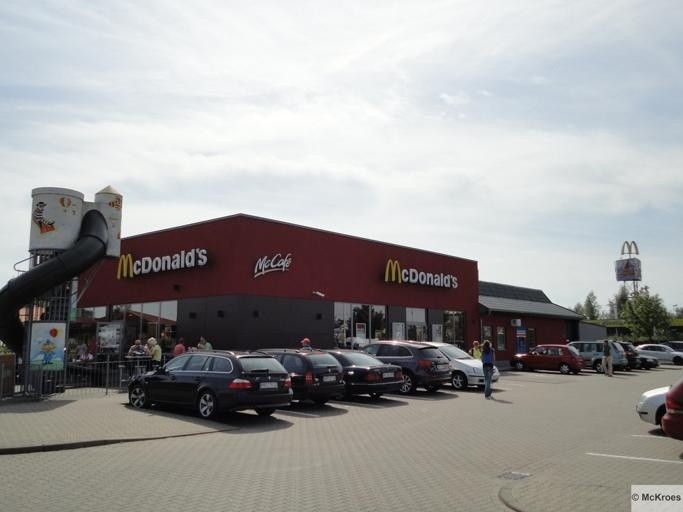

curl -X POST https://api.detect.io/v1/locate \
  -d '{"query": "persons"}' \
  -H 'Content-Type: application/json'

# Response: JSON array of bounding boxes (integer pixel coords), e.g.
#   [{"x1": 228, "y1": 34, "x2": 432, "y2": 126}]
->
[
  {"x1": 33, "y1": 202, "x2": 56, "y2": 229},
  {"x1": 300, "y1": 338, "x2": 313, "y2": 351},
  {"x1": 42, "y1": 338, "x2": 56, "y2": 364},
  {"x1": 469, "y1": 340, "x2": 481, "y2": 358},
  {"x1": 174, "y1": 337, "x2": 185, "y2": 357},
  {"x1": 480, "y1": 341, "x2": 496, "y2": 399},
  {"x1": 602, "y1": 340, "x2": 612, "y2": 377},
  {"x1": 127, "y1": 337, "x2": 161, "y2": 380},
  {"x1": 67, "y1": 335, "x2": 98, "y2": 363},
  {"x1": 197, "y1": 336, "x2": 212, "y2": 350}
]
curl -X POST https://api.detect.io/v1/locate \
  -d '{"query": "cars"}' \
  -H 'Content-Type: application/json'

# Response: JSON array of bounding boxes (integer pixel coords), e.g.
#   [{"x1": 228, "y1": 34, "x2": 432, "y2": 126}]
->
[
  {"x1": 9, "y1": 355, "x2": 102, "y2": 386},
  {"x1": 512, "y1": 344, "x2": 585, "y2": 374},
  {"x1": 636, "y1": 386, "x2": 672, "y2": 426},
  {"x1": 567, "y1": 339, "x2": 682, "y2": 372}
]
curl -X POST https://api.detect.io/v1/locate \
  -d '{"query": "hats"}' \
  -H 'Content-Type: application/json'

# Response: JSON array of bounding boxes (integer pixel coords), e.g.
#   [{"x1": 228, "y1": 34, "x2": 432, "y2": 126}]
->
[{"x1": 300, "y1": 337, "x2": 311, "y2": 343}]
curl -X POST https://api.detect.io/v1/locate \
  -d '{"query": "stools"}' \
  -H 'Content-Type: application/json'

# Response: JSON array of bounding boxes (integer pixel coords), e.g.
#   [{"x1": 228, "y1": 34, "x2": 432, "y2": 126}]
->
[{"x1": 118, "y1": 353, "x2": 160, "y2": 391}]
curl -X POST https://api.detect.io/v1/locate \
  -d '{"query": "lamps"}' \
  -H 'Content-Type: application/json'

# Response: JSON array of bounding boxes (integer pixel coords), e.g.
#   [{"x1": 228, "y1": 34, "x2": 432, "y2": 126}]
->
[{"x1": 311, "y1": 290, "x2": 325, "y2": 298}]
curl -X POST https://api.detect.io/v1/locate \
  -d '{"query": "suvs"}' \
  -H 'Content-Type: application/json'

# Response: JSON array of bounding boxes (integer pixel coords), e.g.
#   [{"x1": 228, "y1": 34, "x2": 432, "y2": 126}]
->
[{"x1": 660, "y1": 377, "x2": 683, "y2": 442}]
